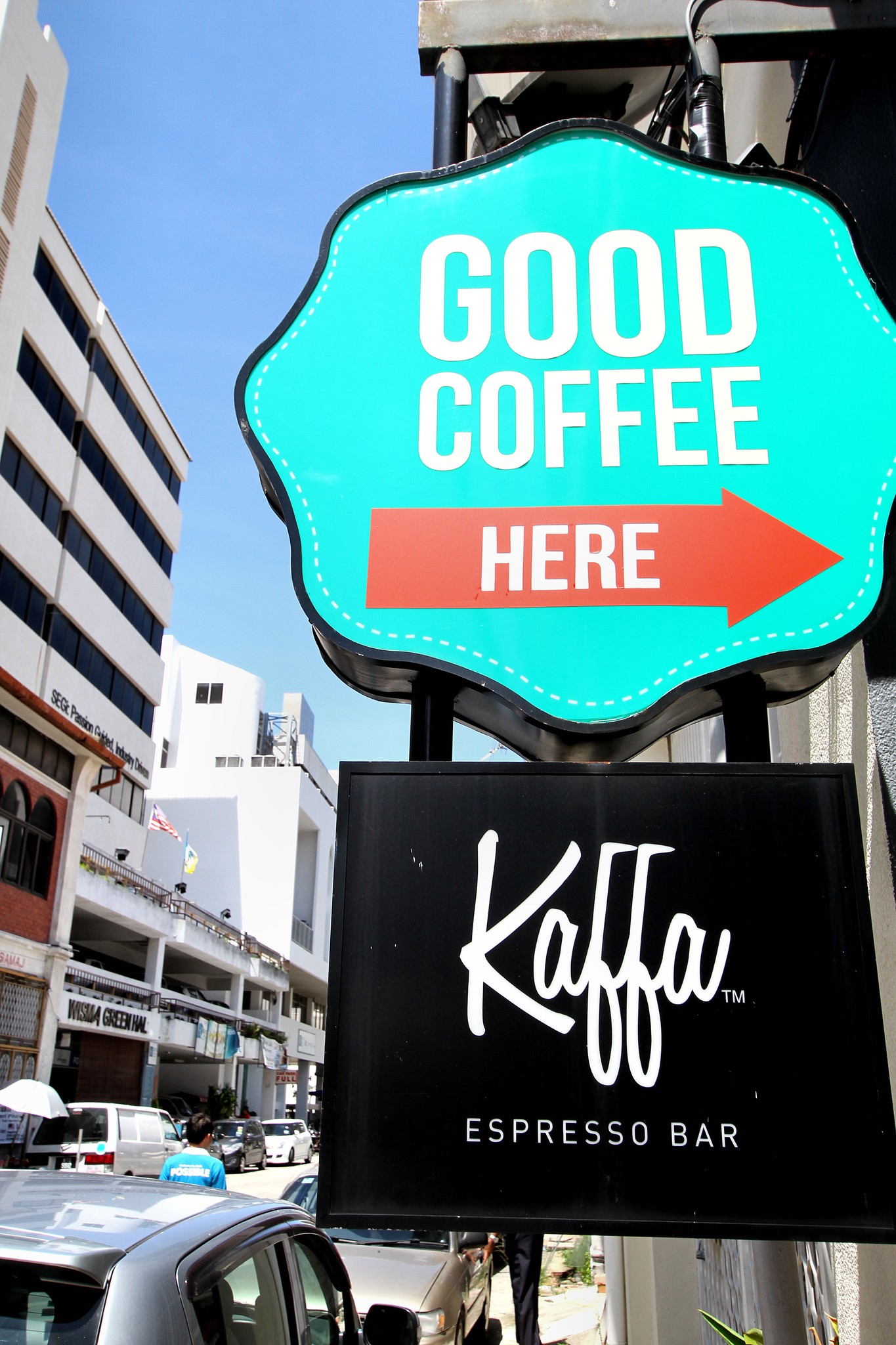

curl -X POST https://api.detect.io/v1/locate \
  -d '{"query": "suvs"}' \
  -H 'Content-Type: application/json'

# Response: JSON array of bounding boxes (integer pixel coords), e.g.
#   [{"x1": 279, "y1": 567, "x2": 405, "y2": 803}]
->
[
  {"x1": 258, "y1": 1119, "x2": 314, "y2": 1164},
  {"x1": 201, "y1": 1117, "x2": 267, "y2": 1174},
  {"x1": 1, "y1": 1164, "x2": 423, "y2": 1345}
]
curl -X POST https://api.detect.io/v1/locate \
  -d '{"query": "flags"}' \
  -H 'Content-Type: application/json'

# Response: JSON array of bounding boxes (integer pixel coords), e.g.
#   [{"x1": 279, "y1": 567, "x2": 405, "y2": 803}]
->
[
  {"x1": 183, "y1": 831, "x2": 198, "y2": 874},
  {"x1": 147, "y1": 803, "x2": 182, "y2": 842}
]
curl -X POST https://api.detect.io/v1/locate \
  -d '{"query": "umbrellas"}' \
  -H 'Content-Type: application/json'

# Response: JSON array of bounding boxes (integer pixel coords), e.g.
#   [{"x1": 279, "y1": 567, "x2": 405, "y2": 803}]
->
[{"x1": 0, "y1": 1079, "x2": 70, "y2": 1168}]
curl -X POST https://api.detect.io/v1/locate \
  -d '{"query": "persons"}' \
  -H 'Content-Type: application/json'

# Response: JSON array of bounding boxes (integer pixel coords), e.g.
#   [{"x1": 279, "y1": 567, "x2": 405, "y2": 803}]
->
[
  {"x1": 461, "y1": 1232, "x2": 544, "y2": 1345},
  {"x1": 234, "y1": 1105, "x2": 250, "y2": 1119},
  {"x1": 158, "y1": 1114, "x2": 227, "y2": 1190}
]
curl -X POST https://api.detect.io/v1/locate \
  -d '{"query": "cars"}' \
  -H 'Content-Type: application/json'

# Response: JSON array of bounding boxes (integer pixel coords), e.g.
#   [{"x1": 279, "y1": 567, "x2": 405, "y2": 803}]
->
[{"x1": 218, "y1": 1152, "x2": 500, "y2": 1345}]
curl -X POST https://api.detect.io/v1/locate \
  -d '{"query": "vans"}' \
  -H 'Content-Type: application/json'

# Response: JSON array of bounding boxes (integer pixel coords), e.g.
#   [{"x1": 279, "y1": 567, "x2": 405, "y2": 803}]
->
[{"x1": 22, "y1": 1102, "x2": 189, "y2": 1183}]
